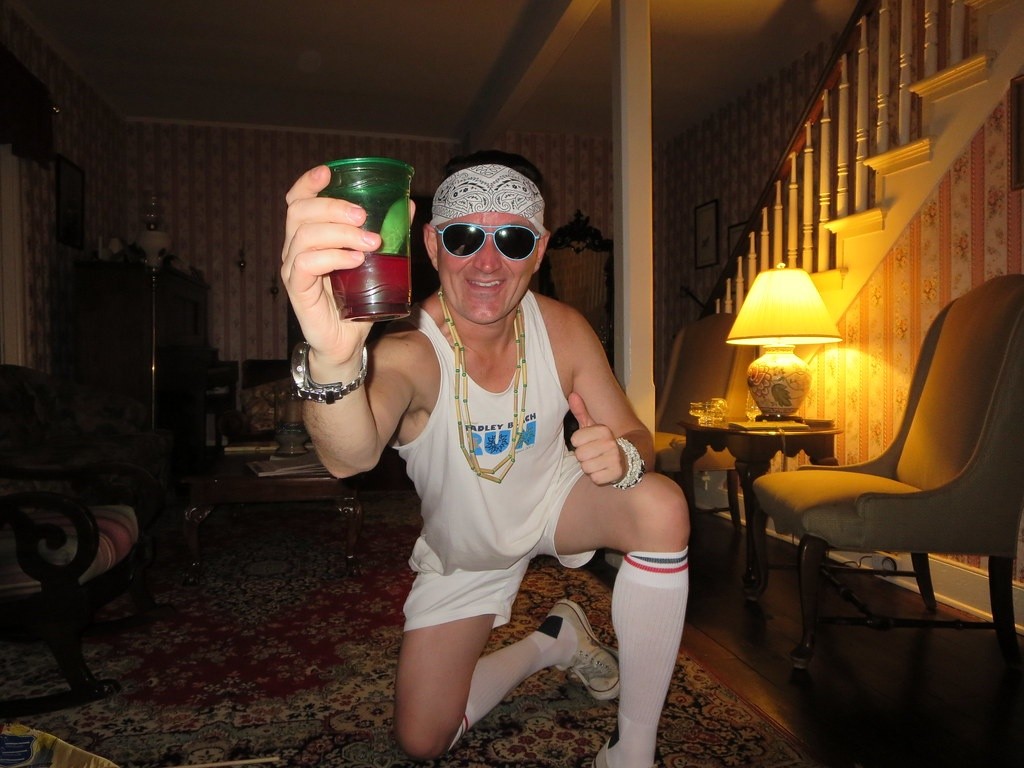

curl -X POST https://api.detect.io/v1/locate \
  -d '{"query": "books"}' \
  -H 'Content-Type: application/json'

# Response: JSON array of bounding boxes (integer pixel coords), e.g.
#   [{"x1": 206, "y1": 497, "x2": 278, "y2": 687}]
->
[
  {"x1": 728, "y1": 421, "x2": 809, "y2": 430},
  {"x1": 247, "y1": 456, "x2": 327, "y2": 477}
]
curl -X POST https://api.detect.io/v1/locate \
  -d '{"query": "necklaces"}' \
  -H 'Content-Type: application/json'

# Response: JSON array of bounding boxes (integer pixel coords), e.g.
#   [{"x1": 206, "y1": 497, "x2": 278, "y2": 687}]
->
[{"x1": 438, "y1": 285, "x2": 527, "y2": 483}]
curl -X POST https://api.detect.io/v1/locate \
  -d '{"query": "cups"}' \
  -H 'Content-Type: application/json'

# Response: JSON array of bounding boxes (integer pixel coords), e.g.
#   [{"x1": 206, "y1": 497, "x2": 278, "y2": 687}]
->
[{"x1": 316, "y1": 157, "x2": 415, "y2": 323}]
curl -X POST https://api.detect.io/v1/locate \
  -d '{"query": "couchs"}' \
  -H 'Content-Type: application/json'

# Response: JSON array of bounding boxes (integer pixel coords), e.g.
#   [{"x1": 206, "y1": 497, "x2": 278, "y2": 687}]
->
[
  {"x1": 0, "y1": 365, "x2": 174, "y2": 528},
  {"x1": 217, "y1": 358, "x2": 311, "y2": 444}
]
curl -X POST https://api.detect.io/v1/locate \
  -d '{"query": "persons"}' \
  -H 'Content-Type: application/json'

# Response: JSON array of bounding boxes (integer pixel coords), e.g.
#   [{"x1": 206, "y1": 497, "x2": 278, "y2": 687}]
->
[{"x1": 280, "y1": 150, "x2": 691, "y2": 768}]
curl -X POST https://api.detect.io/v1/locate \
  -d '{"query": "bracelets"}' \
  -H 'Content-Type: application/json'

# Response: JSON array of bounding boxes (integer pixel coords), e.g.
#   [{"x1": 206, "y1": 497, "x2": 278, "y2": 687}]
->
[{"x1": 613, "y1": 437, "x2": 645, "y2": 489}]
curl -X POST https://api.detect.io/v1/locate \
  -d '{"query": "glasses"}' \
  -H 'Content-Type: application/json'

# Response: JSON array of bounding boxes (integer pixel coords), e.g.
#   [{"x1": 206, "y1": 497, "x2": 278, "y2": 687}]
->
[{"x1": 434, "y1": 223, "x2": 540, "y2": 261}]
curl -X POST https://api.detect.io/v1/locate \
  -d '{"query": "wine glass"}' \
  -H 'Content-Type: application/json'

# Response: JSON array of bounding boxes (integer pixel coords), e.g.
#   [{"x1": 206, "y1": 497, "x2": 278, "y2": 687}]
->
[{"x1": 273, "y1": 377, "x2": 309, "y2": 456}]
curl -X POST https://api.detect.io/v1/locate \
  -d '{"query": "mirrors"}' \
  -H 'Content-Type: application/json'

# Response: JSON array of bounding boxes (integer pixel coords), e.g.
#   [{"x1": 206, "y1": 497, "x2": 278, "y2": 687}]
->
[{"x1": 535, "y1": 209, "x2": 615, "y2": 365}]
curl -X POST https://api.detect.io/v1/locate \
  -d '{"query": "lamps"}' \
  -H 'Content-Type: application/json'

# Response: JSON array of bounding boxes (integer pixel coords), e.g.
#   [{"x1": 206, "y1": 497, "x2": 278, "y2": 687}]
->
[{"x1": 725, "y1": 262, "x2": 841, "y2": 424}]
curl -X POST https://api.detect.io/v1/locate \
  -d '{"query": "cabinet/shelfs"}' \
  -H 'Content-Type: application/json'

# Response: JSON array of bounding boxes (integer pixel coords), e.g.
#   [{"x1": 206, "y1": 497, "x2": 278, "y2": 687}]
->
[
  {"x1": 163, "y1": 343, "x2": 238, "y2": 471},
  {"x1": 72, "y1": 259, "x2": 210, "y2": 431}
]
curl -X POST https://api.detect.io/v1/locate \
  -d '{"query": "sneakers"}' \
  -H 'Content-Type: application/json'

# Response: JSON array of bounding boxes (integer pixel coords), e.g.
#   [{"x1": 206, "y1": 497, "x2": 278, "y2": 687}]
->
[
  {"x1": 546, "y1": 599, "x2": 620, "y2": 700},
  {"x1": 592, "y1": 735, "x2": 619, "y2": 768}
]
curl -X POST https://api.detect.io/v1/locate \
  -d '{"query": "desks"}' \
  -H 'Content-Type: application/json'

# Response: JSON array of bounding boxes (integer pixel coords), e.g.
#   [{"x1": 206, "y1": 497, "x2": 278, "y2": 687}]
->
[{"x1": 672, "y1": 418, "x2": 844, "y2": 586}]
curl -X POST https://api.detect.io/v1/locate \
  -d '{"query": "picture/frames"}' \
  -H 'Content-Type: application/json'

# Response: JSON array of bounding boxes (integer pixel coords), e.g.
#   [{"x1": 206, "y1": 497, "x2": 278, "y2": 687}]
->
[
  {"x1": 52, "y1": 152, "x2": 85, "y2": 248},
  {"x1": 694, "y1": 199, "x2": 720, "y2": 270},
  {"x1": 1009, "y1": 74, "x2": 1024, "y2": 190},
  {"x1": 728, "y1": 221, "x2": 746, "y2": 258}
]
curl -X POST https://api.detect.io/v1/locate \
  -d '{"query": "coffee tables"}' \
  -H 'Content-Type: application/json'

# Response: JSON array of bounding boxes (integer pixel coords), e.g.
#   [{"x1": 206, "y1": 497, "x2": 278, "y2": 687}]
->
[{"x1": 185, "y1": 443, "x2": 367, "y2": 579}]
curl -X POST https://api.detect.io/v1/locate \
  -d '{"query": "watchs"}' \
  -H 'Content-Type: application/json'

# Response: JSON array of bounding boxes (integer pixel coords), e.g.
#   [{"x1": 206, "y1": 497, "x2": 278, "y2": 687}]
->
[{"x1": 290, "y1": 341, "x2": 368, "y2": 404}]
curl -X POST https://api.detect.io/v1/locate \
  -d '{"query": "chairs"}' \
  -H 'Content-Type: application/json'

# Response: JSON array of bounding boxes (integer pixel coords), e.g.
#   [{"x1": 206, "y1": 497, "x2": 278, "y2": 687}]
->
[
  {"x1": 743, "y1": 274, "x2": 1024, "y2": 669},
  {"x1": 654, "y1": 313, "x2": 760, "y2": 532},
  {"x1": 0, "y1": 464, "x2": 177, "y2": 719}
]
